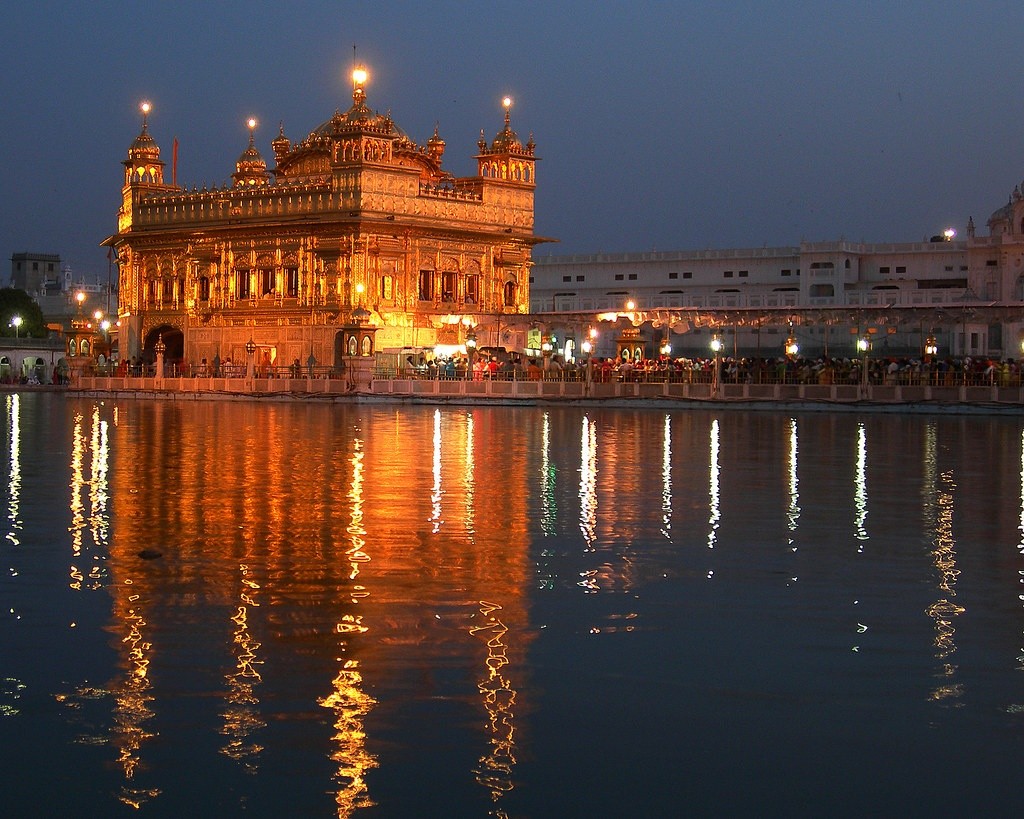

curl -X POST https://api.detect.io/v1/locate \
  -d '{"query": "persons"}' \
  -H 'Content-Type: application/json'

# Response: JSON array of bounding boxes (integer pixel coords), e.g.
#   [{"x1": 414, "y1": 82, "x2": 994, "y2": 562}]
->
[
  {"x1": 219, "y1": 357, "x2": 233, "y2": 378},
  {"x1": 53, "y1": 364, "x2": 69, "y2": 386},
  {"x1": 290, "y1": 358, "x2": 302, "y2": 379},
  {"x1": 562, "y1": 354, "x2": 1024, "y2": 386},
  {"x1": 405, "y1": 355, "x2": 566, "y2": 382},
  {"x1": 196, "y1": 359, "x2": 209, "y2": 378},
  {"x1": 0, "y1": 374, "x2": 41, "y2": 386},
  {"x1": 109, "y1": 355, "x2": 191, "y2": 378}
]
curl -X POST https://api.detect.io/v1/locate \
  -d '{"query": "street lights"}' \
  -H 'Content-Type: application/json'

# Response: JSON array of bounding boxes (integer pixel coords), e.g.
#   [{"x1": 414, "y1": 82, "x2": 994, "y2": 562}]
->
[
  {"x1": 584, "y1": 340, "x2": 596, "y2": 397},
  {"x1": 155, "y1": 333, "x2": 167, "y2": 378},
  {"x1": 464, "y1": 325, "x2": 479, "y2": 381},
  {"x1": 14, "y1": 318, "x2": 21, "y2": 338},
  {"x1": 859, "y1": 334, "x2": 873, "y2": 400},
  {"x1": 711, "y1": 340, "x2": 723, "y2": 400},
  {"x1": 242, "y1": 335, "x2": 257, "y2": 379}
]
